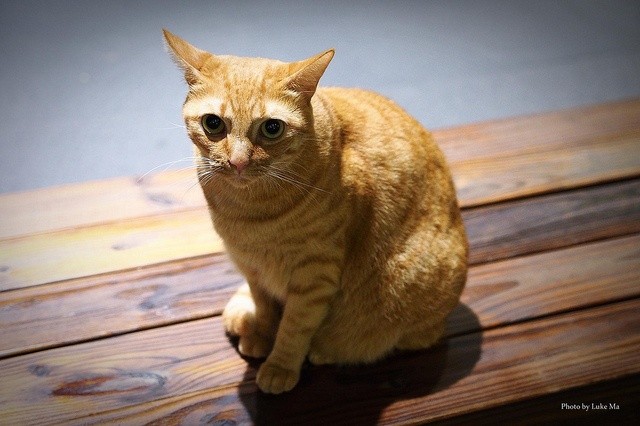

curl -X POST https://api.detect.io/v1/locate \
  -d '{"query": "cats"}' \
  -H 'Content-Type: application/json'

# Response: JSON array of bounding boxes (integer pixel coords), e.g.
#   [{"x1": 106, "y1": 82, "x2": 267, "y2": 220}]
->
[{"x1": 159, "y1": 23, "x2": 471, "y2": 396}]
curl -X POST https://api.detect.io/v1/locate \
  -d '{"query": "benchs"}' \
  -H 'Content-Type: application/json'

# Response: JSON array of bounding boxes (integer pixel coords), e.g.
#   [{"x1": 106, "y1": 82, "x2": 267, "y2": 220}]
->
[{"x1": 0, "y1": 101, "x2": 640, "y2": 426}]
